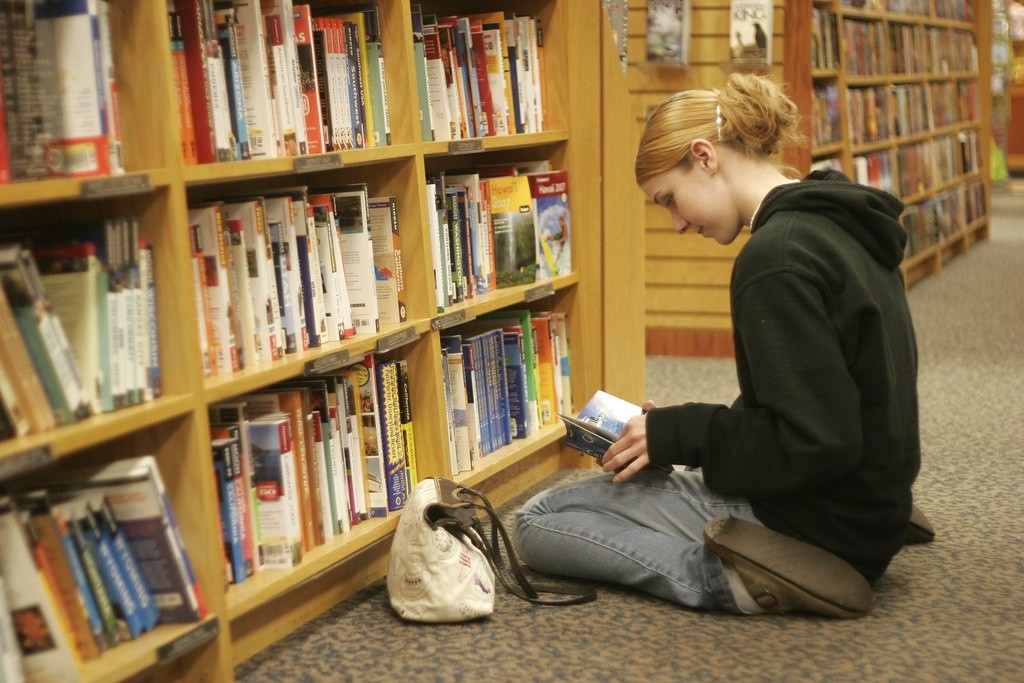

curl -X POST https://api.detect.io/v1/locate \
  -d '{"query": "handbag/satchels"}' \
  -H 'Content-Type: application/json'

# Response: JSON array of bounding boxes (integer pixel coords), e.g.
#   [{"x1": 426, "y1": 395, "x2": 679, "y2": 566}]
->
[{"x1": 386, "y1": 476, "x2": 598, "y2": 624}]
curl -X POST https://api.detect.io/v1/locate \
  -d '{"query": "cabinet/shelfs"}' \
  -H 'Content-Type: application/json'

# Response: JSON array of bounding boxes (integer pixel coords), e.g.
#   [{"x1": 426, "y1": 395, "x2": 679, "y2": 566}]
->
[
  {"x1": 628, "y1": 1, "x2": 992, "y2": 362},
  {"x1": 1, "y1": 1, "x2": 602, "y2": 683}
]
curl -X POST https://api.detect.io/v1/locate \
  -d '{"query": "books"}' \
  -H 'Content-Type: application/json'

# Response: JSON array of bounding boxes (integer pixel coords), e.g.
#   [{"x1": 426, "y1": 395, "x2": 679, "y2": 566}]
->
[
  {"x1": 729, "y1": 0, "x2": 773, "y2": 68},
  {"x1": 645, "y1": 0, "x2": 689, "y2": 69},
  {"x1": 607, "y1": 0, "x2": 628, "y2": 78},
  {"x1": 810, "y1": 0, "x2": 986, "y2": 264},
  {"x1": 554, "y1": 389, "x2": 649, "y2": 476},
  {"x1": 0, "y1": 0, "x2": 575, "y2": 683}
]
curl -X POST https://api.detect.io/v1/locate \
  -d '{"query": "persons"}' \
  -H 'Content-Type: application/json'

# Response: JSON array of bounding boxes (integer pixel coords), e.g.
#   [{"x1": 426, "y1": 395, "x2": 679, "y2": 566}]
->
[{"x1": 511, "y1": 76, "x2": 935, "y2": 620}]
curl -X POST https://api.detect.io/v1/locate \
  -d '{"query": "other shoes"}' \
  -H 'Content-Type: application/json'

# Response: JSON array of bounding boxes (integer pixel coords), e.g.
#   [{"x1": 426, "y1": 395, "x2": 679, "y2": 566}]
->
[
  {"x1": 904, "y1": 503, "x2": 936, "y2": 542},
  {"x1": 701, "y1": 515, "x2": 876, "y2": 620}
]
